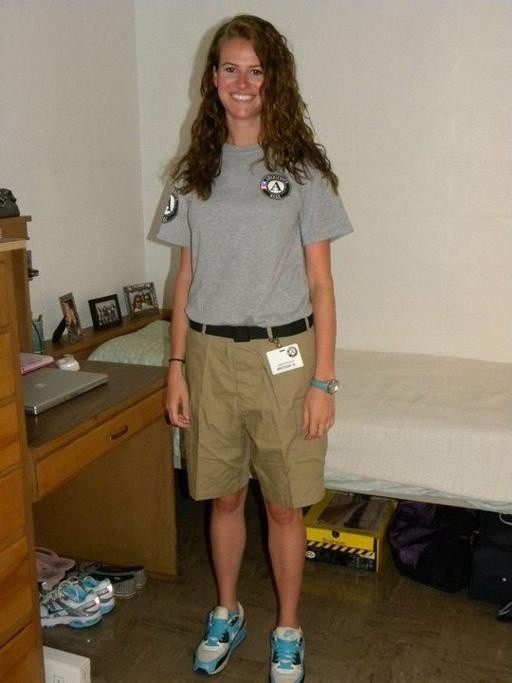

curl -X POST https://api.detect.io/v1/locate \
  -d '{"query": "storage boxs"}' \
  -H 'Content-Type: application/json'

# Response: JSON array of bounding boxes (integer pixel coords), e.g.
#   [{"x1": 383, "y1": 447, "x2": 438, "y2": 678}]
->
[{"x1": 303, "y1": 489, "x2": 399, "y2": 573}]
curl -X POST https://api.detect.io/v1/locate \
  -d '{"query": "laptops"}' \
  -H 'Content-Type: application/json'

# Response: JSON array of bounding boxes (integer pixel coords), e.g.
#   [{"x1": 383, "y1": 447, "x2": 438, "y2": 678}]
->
[{"x1": 20, "y1": 366, "x2": 110, "y2": 417}]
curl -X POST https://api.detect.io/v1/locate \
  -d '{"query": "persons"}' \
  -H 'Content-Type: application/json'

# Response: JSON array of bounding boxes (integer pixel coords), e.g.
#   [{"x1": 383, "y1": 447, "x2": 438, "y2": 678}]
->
[{"x1": 156, "y1": 15, "x2": 354, "y2": 683}]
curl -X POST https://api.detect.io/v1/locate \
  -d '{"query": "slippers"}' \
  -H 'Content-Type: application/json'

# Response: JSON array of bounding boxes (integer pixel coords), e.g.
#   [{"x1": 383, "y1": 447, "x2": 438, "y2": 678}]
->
[{"x1": 36, "y1": 546, "x2": 76, "y2": 583}]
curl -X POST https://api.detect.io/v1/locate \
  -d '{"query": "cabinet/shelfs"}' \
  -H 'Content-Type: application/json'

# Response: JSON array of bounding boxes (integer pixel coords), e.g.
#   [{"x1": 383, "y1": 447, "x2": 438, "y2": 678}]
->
[{"x1": 1, "y1": 215, "x2": 180, "y2": 683}]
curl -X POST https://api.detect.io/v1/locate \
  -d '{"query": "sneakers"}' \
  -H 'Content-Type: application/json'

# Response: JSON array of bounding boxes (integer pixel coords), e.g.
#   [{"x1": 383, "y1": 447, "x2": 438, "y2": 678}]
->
[
  {"x1": 192, "y1": 601, "x2": 248, "y2": 676},
  {"x1": 269, "y1": 624, "x2": 305, "y2": 683},
  {"x1": 68, "y1": 560, "x2": 148, "y2": 600},
  {"x1": 39, "y1": 575, "x2": 116, "y2": 630}
]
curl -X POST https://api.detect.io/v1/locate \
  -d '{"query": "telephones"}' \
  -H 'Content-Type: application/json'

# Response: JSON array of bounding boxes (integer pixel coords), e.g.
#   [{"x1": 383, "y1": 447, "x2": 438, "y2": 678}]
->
[{"x1": 0, "y1": 188, "x2": 20, "y2": 217}]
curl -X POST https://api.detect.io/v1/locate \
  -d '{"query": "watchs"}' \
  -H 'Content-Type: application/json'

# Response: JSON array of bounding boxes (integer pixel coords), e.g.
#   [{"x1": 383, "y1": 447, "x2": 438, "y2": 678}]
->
[{"x1": 310, "y1": 377, "x2": 340, "y2": 395}]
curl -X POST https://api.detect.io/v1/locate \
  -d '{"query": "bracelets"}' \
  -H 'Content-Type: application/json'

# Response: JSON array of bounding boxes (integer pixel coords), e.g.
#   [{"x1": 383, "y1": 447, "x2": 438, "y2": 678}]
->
[{"x1": 169, "y1": 358, "x2": 187, "y2": 362}]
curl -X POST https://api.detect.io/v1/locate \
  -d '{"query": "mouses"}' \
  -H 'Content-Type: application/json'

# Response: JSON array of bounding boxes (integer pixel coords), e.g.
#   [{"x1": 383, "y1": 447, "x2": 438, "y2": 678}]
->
[{"x1": 55, "y1": 357, "x2": 81, "y2": 372}]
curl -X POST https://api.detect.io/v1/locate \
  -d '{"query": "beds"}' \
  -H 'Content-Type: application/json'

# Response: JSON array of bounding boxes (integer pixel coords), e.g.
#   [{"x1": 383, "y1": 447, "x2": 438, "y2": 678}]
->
[{"x1": 39, "y1": 309, "x2": 511, "y2": 515}]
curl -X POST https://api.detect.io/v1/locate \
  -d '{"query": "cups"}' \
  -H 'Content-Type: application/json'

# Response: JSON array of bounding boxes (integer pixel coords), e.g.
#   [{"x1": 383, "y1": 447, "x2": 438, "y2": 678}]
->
[{"x1": 31, "y1": 318, "x2": 45, "y2": 355}]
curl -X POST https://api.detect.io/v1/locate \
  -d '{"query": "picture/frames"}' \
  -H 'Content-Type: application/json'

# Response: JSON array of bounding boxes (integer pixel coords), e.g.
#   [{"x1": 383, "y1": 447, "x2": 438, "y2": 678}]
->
[
  {"x1": 89, "y1": 293, "x2": 123, "y2": 331},
  {"x1": 122, "y1": 281, "x2": 160, "y2": 320},
  {"x1": 59, "y1": 292, "x2": 84, "y2": 344}
]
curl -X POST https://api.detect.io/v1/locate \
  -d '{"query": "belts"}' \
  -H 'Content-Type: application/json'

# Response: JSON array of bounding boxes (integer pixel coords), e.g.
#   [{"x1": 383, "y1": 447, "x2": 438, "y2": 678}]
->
[{"x1": 189, "y1": 313, "x2": 314, "y2": 342}]
